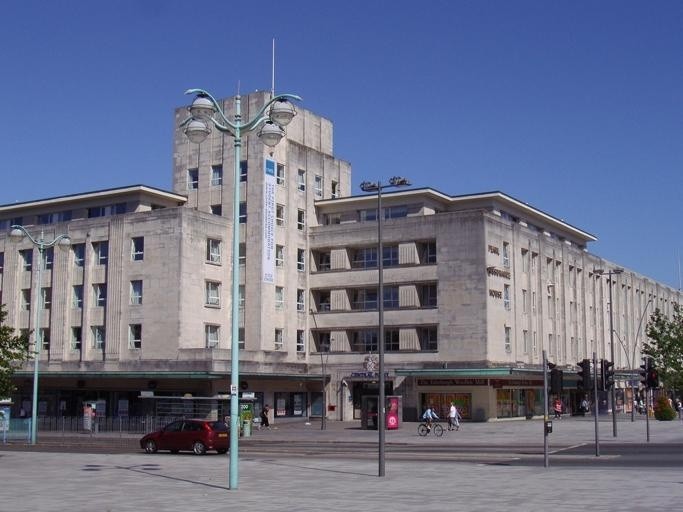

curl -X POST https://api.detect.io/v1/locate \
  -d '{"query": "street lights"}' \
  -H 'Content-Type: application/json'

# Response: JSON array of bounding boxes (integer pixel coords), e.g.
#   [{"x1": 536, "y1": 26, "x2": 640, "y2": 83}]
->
[
  {"x1": 178, "y1": 81, "x2": 304, "y2": 490},
  {"x1": 309, "y1": 307, "x2": 336, "y2": 430},
  {"x1": 613, "y1": 299, "x2": 654, "y2": 422},
  {"x1": 11, "y1": 225, "x2": 72, "y2": 446},
  {"x1": 593, "y1": 268, "x2": 624, "y2": 437},
  {"x1": 359, "y1": 176, "x2": 412, "y2": 477}
]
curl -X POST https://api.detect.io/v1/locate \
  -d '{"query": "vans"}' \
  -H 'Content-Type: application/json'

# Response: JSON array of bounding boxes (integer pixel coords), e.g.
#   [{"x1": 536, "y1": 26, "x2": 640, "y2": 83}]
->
[{"x1": 140, "y1": 419, "x2": 230, "y2": 456}]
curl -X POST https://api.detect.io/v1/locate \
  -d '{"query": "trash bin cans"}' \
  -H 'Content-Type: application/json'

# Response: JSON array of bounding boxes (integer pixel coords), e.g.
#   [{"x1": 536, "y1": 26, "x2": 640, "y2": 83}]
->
[{"x1": 242, "y1": 420, "x2": 251, "y2": 439}]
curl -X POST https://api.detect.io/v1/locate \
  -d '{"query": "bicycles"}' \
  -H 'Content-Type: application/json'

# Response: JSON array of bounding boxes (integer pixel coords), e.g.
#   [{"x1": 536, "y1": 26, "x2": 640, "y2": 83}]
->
[{"x1": 418, "y1": 418, "x2": 443, "y2": 437}]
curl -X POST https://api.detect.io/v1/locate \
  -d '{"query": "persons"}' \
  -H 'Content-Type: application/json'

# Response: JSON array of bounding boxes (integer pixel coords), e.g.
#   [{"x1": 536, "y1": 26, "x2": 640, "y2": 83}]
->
[
  {"x1": 454, "y1": 407, "x2": 463, "y2": 431},
  {"x1": 553, "y1": 398, "x2": 562, "y2": 419},
  {"x1": 259, "y1": 404, "x2": 272, "y2": 430},
  {"x1": 447, "y1": 401, "x2": 459, "y2": 431},
  {"x1": 579, "y1": 398, "x2": 588, "y2": 416},
  {"x1": 422, "y1": 405, "x2": 439, "y2": 436},
  {"x1": 632, "y1": 398, "x2": 682, "y2": 419}
]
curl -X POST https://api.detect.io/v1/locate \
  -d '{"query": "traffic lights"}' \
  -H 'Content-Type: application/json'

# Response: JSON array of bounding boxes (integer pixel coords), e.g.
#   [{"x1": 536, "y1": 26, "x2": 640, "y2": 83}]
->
[
  {"x1": 648, "y1": 358, "x2": 659, "y2": 387},
  {"x1": 577, "y1": 359, "x2": 591, "y2": 393},
  {"x1": 640, "y1": 356, "x2": 649, "y2": 388},
  {"x1": 601, "y1": 359, "x2": 615, "y2": 391}
]
